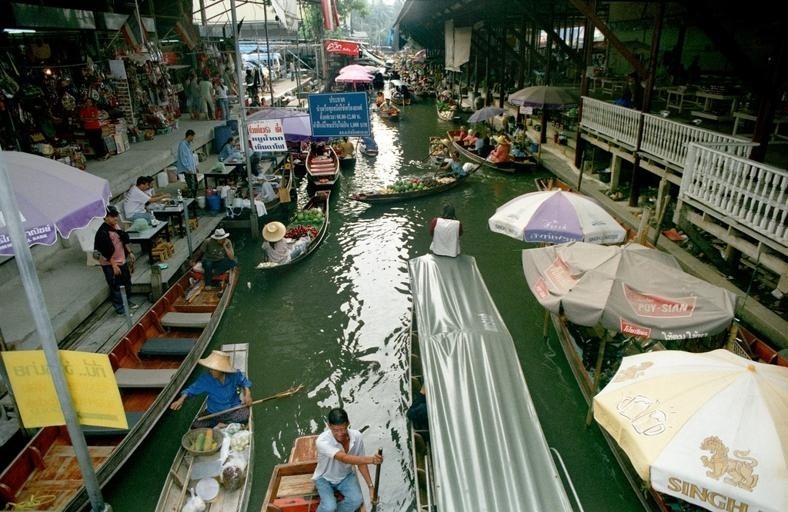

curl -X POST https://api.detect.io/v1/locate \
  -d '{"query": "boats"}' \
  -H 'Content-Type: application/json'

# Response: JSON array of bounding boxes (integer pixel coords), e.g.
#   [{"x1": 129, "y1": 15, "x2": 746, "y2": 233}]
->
[
  {"x1": 534, "y1": 174, "x2": 659, "y2": 255},
  {"x1": 401, "y1": 250, "x2": 577, "y2": 512}
]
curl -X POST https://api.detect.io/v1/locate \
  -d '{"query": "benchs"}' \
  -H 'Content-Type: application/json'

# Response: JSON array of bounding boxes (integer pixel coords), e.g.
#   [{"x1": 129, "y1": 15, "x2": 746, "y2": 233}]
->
[
  {"x1": 113, "y1": 368, "x2": 179, "y2": 388},
  {"x1": 310, "y1": 159, "x2": 335, "y2": 172},
  {"x1": 160, "y1": 312, "x2": 212, "y2": 329},
  {"x1": 177, "y1": 173, "x2": 204, "y2": 188},
  {"x1": 113, "y1": 200, "x2": 134, "y2": 231},
  {"x1": 80, "y1": 412, "x2": 144, "y2": 435},
  {"x1": 139, "y1": 337, "x2": 194, "y2": 356},
  {"x1": 75, "y1": 217, "x2": 105, "y2": 267}
]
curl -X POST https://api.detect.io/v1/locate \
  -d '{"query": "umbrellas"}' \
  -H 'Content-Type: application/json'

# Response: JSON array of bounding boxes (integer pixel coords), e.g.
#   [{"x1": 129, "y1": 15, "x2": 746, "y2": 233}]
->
[
  {"x1": 339, "y1": 64, "x2": 372, "y2": 74},
  {"x1": 522, "y1": 237, "x2": 740, "y2": 340},
  {"x1": 590, "y1": 347, "x2": 787, "y2": 512},
  {"x1": 243, "y1": 106, "x2": 330, "y2": 143},
  {"x1": 0, "y1": 149, "x2": 114, "y2": 258},
  {"x1": 467, "y1": 103, "x2": 510, "y2": 133},
  {"x1": 336, "y1": 68, "x2": 373, "y2": 92},
  {"x1": 487, "y1": 187, "x2": 629, "y2": 244},
  {"x1": 509, "y1": 84, "x2": 581, "y2": 162}
]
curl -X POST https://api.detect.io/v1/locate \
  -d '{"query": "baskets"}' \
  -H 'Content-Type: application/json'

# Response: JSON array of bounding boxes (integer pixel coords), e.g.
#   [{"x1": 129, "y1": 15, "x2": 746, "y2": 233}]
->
[{"x1": 181, "y1": 427, "x2": 224, "y2": 455}]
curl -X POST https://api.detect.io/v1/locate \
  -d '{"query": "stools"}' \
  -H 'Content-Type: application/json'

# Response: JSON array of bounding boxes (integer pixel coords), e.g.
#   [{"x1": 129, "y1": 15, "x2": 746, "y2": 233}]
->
[
  {"x1": 152, "y1": 237, "x2": 175, "y2": 262},
  {"x1": 189, "y1": 218, "x2": 197, "y2": 230},
  {"x1": 551, "y1": 120, "x2": 568, "y2": 131}
]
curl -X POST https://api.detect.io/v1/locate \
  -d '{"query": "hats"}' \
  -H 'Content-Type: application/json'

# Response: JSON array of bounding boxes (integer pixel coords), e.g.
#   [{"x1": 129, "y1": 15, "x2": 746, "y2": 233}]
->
[
  {"x1": 262, "y1": 221, "x2": 287, "y2": 242},
  {"x1": 106, "y1": 205, "x2": 118, "y2": 215},
  {"x1": 211, "y1": 228, "x2": 230, "y2": 240},
  {"x1": 199, "y1": 349, "x2": 239, "y2": 374},
  {"x1": 492, "y1": 134, "x2": 507, "y2": 145}
]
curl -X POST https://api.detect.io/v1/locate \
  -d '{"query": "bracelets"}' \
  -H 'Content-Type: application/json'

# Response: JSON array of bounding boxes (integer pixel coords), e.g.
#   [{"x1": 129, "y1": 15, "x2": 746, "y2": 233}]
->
[
  {"x1": 367, "y1": 483, "x2": 373, "y2": 487},
  {"x1": 128, "y1": 250, "x2": 133, "y2": 255}
]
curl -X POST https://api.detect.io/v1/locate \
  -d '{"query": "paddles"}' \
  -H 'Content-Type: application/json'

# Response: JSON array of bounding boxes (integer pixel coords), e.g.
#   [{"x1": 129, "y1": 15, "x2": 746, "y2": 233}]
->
[{"x1": 198, "y1": 386, "x2": 298, "y2": 421}]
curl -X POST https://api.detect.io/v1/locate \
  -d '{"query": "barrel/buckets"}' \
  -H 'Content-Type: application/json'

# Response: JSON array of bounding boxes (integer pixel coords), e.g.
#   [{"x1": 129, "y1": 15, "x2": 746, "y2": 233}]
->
[
  {"x1": 207, "y1": 194, "x2": 221, "y2": 211},
  {"x1": 194, "y1": 476, "x2": 222, "y2": 504}
]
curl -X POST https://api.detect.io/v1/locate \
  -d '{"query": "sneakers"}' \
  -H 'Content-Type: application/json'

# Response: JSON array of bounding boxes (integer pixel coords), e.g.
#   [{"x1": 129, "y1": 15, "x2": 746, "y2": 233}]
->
[
  {"x1": 127, "y1": 300, "x2": 139, "y2": 310},
  {"x1": 115, "y1": 305, "x2": 126, "y2": 315}
]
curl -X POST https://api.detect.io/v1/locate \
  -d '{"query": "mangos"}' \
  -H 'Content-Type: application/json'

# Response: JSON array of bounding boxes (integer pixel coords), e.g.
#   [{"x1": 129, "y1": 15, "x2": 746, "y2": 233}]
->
[{"x1": 195, "y1": 429, "x2": 215, "y2": 451}]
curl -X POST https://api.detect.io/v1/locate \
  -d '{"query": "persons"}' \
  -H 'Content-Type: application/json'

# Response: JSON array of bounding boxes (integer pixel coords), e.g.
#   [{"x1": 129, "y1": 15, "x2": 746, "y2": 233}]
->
[
  {"x1": 375, "y1": 91, "x2": 383, "y2": 107},
  {"x1": 175, "y1": 130, "x2": 199, "y2": 197},
  {"x1": 78, "y1": 97, "x2": 111, "y2": 162},
  {"x1": 189, "y1": 227, "x2": 240, "y2": 292},
  {"x1": 260, "y1": 221, "x2": 305, "y2": 265},
  {"x1": 184, "y1": 67, "x2": 267, "y2": 121},
  {"x1": 618, "y1": 70, "x2": 648, "y2": 114},
  {"x1": 144, "y1": 175, "x2": 168, "y2": 211},
  {"x1": 429, "y1": 204, "x2": 465, "y2": 258},
  {"x1": 217, "y1": 136, "x2": 236, "y2": 161},
  {"x1": 91, "y1": 205, "x2": 138, "y2": 316},
  {"x1": 254, "y1": 172, "x2": 278, "y2": 202},
  {"x1": 341, "y1": 137, "x2": 354, "y2": 156},
  {"x1": 309, "y1": 408, "x2": 383, "y2": 512},
  {"x1": 123, "y1": 176, "x2": 172, "y2": 226},
  {"x1": 169, "y1": 349, "x2": 255, "y2": 429},
  {"x1": 438, "y1": 151, "x2": 467, "y2": 178},
  {"x1": 379, "y1": 98, "x2": 401, "y2": 113},
  {"x1": 459, "y1": 125, "x2": 512, "y2": 163}
]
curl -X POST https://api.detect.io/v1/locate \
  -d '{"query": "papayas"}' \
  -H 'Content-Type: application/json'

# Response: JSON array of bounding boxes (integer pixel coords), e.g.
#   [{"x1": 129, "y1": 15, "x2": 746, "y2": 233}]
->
[
  {"x1": 290, "y1": 207, "x2": 324, "y2": 225},
  {"x1": 387, "y1": 180, "x2": 424, "y2": 194}
]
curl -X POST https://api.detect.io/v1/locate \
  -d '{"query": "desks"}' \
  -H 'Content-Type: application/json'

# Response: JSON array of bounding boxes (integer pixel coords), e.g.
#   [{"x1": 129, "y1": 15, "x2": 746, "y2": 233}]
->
[
  {"x1": 147, "y1": 197, "x2": 197, "y2": 238},
  {"x1": 222, "y1": 151, "x2": 256, "y2": 180},
  {"x1": 205, "y1": 166, "x2": 236, "y2": 190},
  {"x1": 125, "y1": 220, "x2": 170, "y2": 265},
  {"x1": 579, "y1": 72, "x2": 788, "y2": 145}
]
